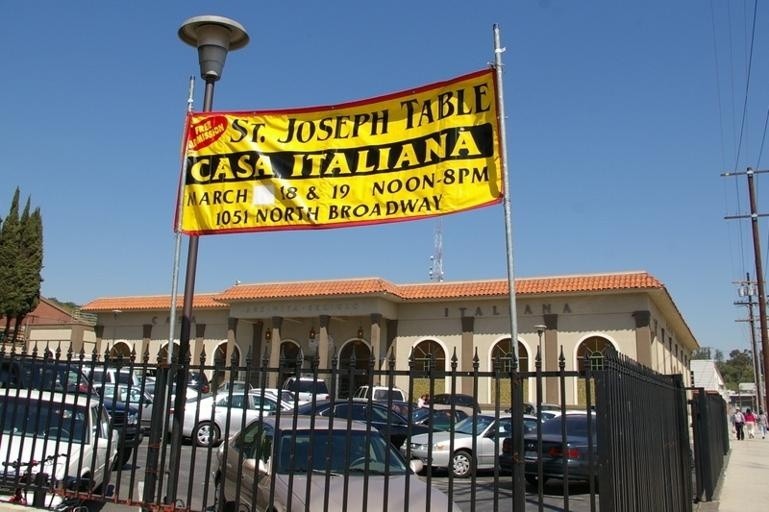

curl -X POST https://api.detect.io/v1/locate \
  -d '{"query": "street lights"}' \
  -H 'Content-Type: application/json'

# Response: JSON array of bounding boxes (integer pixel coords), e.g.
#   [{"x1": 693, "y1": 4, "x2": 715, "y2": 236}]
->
[
  {"x1": 110, "y1": 309, "x2": 124, "y2": 355},
  {"x1": 536, "y1": 321, "x2": 547, "y2": 398},
  {"x1": 738, "y1": 272, "x2": 764, "y2": 418},
  {"x1": 161, "y1": 11, "x2": 250, "y2": 503}
]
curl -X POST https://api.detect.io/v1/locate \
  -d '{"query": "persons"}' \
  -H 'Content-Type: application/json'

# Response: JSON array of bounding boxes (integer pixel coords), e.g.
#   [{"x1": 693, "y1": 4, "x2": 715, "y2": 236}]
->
[
  {"x1": 416, "y1": 393, "x2": 428, "y2": 408},
  {"x1": 731, "y1": 406, "x2": 769, "y2": 441}
]
